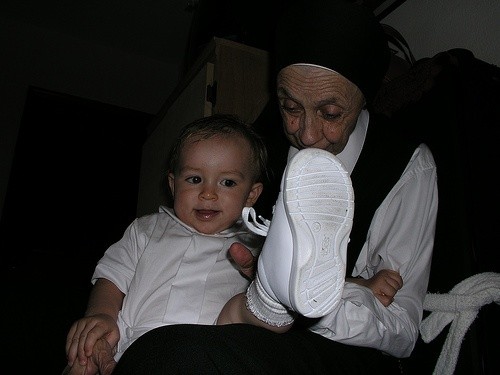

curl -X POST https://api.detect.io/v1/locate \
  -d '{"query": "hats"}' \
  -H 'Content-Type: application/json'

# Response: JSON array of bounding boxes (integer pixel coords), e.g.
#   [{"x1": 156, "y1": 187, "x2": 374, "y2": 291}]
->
[{"x1": 268, "y1": 1, "x2": 392, "y2": 105}]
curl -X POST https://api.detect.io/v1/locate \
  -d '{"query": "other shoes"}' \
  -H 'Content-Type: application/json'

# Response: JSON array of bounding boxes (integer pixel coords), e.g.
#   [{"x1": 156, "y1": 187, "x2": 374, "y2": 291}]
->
[{"x1": 256, "y1": 147, "x2": 354, "y2": 319}]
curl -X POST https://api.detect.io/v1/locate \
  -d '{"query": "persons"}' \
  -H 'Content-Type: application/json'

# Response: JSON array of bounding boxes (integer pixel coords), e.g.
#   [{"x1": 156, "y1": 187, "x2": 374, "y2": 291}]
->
[
  {"x1": 62, "y1": 0, "x2": 440, "y2": 375},
  {"x1": 63, "y1": 110, "x2": 405, "y2": 367}
]
